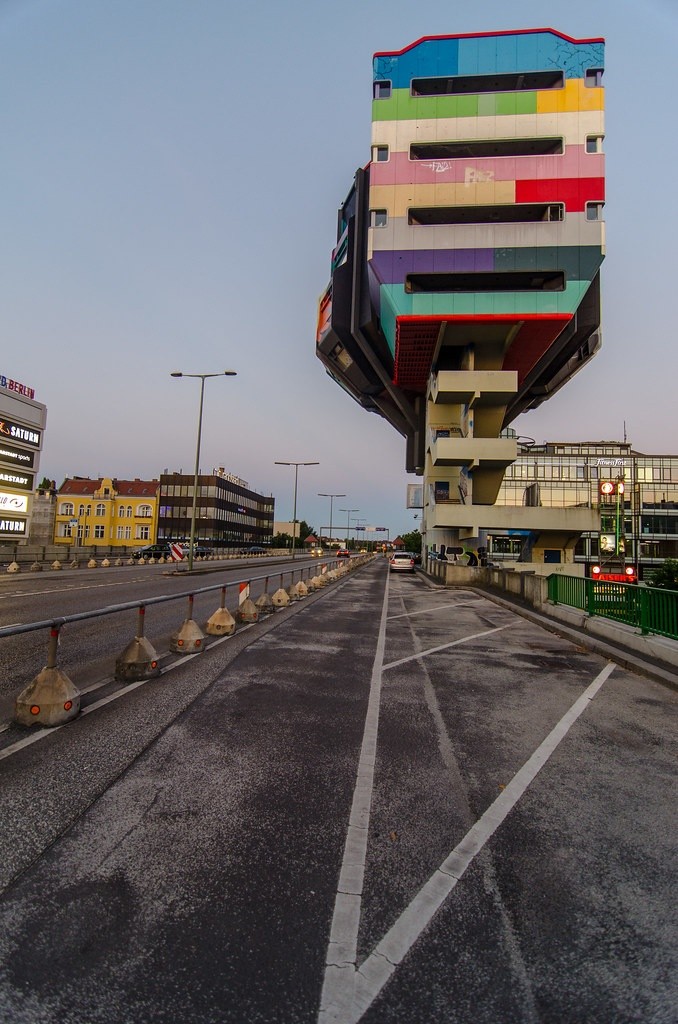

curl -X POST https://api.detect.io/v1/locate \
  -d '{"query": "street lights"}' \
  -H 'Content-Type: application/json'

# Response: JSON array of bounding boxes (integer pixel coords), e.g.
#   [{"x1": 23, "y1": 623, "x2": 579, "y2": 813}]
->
[
  {"x1": 170, "y1": 369, "x2": 238, "y2": 572},
  {"x1": 275, "y1": 461, "x2": 321, "y2": 560},
  {"x1": 317, "y1": 493, "x2": 347, "y2": 555},
  {"x1": 338, "y1": 509, "x2": 360, "y2": 549}
]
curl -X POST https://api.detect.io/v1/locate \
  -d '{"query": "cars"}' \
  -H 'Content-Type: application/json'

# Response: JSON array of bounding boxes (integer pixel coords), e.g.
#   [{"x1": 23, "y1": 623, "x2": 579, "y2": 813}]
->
[
  {"x1": 388, "y1": 552, "x2": 416, "y2": 574},
  {"x1": 180, "y1": 543, "x2": 196, "y2": 558},
  {"x1": 240, "y1": 546, "x2": 267, "y2": 554},
  {"x1": 195, "y1": 546, "x2": 214, "y2": 557},
  {"x1": 312, "y1": 547, "x2": 323, "y2": 555},
  {"x1": 337, "y1": 548, "x2": 350, "y2": 557},
  {"x1": 132, "y1": 544, "x2": 172, "y2": 559}
]
